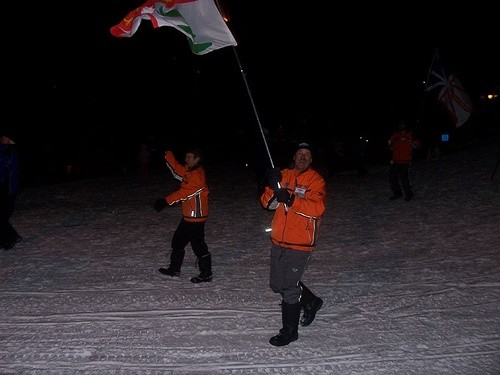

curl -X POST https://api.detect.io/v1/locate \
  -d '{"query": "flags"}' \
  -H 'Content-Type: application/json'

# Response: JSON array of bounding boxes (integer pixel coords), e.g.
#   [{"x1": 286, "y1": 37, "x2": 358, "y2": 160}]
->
[
  {"x1": 110, "y1": 0, "x2": 238, "y2": 56},
  {"x1": 423, "y1": 54, "x2": 473, "y2": 128}
]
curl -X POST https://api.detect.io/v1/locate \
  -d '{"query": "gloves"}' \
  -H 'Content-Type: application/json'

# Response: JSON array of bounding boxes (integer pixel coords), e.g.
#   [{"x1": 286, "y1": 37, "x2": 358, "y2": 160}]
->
[
  {"x1": 163, "y1": 140, "x2": 172, "y2": 150},
  {"x1": 275, "y1": 188, "x2": 295, "y2": 207},
  {"x1": 155, "y1": 198, "x2": 168, "y2": 212},
  {"x1": 267, "y1": 164, "x2": 282, "y2": 187}
]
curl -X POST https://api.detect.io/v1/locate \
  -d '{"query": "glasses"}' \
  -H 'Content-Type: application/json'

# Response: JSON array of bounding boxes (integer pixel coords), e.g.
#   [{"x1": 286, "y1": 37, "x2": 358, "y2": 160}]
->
[{"x1": 185, "y1": 156, "x2": 193, "y2": 159}]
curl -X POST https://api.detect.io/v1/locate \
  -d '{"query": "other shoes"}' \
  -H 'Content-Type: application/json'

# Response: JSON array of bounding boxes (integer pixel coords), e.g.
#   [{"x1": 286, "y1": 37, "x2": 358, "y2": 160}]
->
[
  {"x1": 390, "y1": 193, "x2": 403, "y2": 200},
  {"x1": 5, "y1": 235, "x2": 23, "y2": 250},
  {"x1": 404, "y1": 196, "x2": 415, "y2": 201}
]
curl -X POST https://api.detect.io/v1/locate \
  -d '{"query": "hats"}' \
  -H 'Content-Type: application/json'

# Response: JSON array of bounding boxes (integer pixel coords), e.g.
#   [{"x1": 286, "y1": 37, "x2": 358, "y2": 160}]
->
[{"x1": 294, "y1": 142, "x2": 312, "y2": 154}]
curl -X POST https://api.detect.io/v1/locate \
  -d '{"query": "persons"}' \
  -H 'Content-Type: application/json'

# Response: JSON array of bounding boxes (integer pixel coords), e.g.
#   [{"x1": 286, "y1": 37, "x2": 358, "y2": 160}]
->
[
  {"x1": 260, "y1": 142, "x2": 326, "y2": 346},
  {"x1": 140, "y1": 144, "x2": 150, "y2": 175},
  {"x1": 261, "y1": 118, "x2": 330, "y2": 187},
  {"x1": 154, "y1": 148, "x2": 212, "y2": 283},
  {"x1": 384, "y1": 119, "x2": 421, "y2": 201},
  {"x1": 0, "y1": 135, "x2": 22, "y2": 250}
]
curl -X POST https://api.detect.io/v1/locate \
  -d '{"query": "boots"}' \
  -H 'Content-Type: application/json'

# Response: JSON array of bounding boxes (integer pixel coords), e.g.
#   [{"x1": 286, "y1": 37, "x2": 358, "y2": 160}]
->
[
  {"x1": 159, "y1": 250, "x2": 185, "y2": 276},
  {"x1": 298, "y1": 280, "x2": 323, "y2": 327},
  {"x1": 191, "y1": 252, "x2": 213, "y2": 283},
  {"x1": 269, "y1": 300, "x2": 301, "y2": 346}
]
